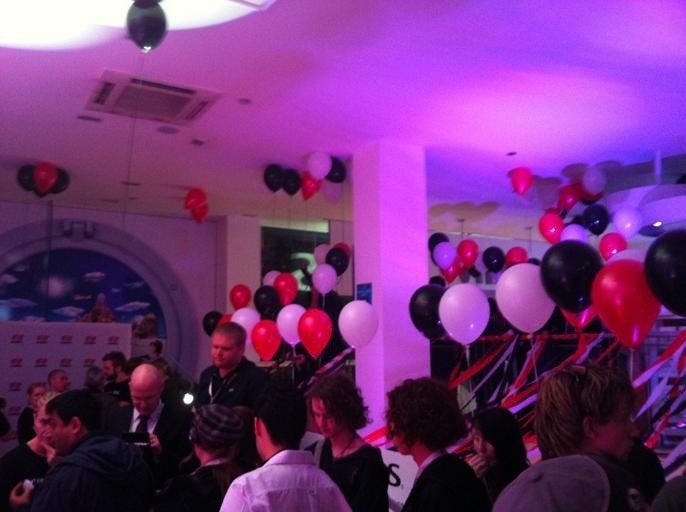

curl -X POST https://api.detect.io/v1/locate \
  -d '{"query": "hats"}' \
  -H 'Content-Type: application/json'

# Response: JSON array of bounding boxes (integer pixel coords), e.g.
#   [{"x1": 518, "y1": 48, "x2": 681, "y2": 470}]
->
[
  {"x1": 491, "y1": 455, "x2": 611, "y2": 512},
  {"x1": 191, "y1": 404, "x2": 238, "y2": 450}
]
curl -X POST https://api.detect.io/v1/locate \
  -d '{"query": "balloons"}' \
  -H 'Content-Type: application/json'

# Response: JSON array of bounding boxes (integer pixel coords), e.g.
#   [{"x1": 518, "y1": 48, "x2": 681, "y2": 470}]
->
[
  {"x1": 125, "y1": 3, "x2": 171, "y2": 53},
  {"x1": 13, "y1": 158, "x2": 72, "y2": 201},
  {"x1": 180, "y1": 149, "x2": 686, "y2": 365}
]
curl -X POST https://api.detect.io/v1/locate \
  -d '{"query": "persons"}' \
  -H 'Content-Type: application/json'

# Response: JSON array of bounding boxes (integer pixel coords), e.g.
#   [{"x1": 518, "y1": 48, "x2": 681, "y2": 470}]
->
[
  {"x1": 491, "y1": 360, "x2": 686, "y2": 511},
  {"x1": 299, "y1": 369, "x2": 390, "y2": 511},
  {"x1": 463, "y1": 407, "x2": 529, "y2": 505},
  {"x1": 217, "y1": 375, "x2": 354, "y2": 512},
  {"x1": 103, "y1": 363, "x2": 199, "y2": 477},
  {"x1": 167, "y1": 402, "x2": 251, "y2": 511},
  {"x1": 383, "y1": 375, "x2": 493, "y2": 511},
  {"x1": 0, "y1": 339, "x2": 201, "y2": 511},
  {"x1": 11, "y1": 386, "x2": 159, "y2": 511},
  {"x1": 190, "y1": 319, "x2": 267, "y2": 475}
]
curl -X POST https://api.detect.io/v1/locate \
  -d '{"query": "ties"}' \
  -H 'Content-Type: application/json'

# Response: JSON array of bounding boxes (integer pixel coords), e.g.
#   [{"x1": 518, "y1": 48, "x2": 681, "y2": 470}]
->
[{"x1": 136, "y1": 414, "x2": 150, "y2": 431}]
